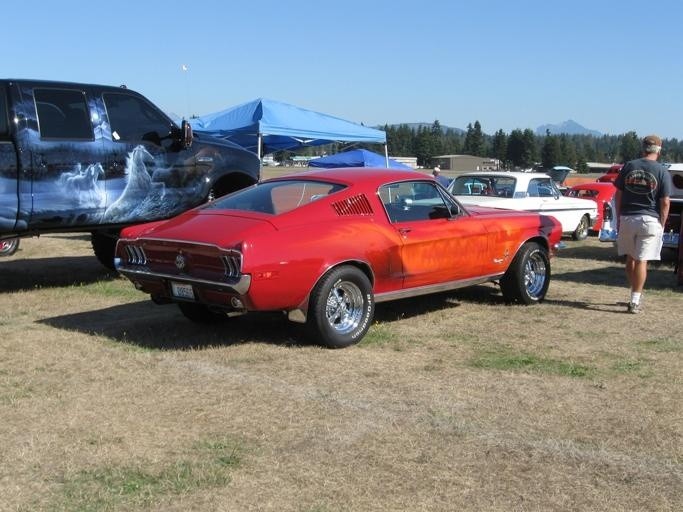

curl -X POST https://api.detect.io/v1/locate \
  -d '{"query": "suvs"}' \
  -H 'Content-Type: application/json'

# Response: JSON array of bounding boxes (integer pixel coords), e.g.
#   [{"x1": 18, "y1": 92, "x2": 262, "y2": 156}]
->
[{"x1": 0, "y1": 77, "x2": 261, "y2": 272}]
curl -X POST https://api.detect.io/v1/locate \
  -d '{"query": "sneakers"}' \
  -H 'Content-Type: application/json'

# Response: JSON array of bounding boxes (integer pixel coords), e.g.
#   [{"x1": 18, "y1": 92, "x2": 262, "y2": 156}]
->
[{"x1": 628, "y1": 302, "x2": 639, "y2": 314}]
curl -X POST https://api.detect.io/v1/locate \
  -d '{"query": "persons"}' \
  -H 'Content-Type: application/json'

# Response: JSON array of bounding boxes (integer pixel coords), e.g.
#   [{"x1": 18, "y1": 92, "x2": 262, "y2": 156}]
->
[
  {"x1": 431, "y1": 165, "x2": 450, "y2": 189},
  {"x1": 613, "y1": 135, "x2": 673, "y2": 314}
]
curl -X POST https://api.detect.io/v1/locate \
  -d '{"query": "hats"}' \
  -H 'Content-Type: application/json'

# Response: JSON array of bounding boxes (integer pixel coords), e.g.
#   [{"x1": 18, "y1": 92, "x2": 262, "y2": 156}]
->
[{"x1": 642, "y1": 136, "x2": 662, "y2": 146}]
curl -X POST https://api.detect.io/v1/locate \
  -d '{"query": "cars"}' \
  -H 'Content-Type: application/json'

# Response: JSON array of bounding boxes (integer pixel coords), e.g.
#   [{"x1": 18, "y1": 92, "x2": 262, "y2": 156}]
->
[
  {"x1": 405, "y1": 172, "x2": 597, "y2": 241},
  {"x1": 111, "y1": 163, "x2": 563, "y2": 349},
  {"x1": 564, "y1": 161, "x2": 682, "y2": 260}
]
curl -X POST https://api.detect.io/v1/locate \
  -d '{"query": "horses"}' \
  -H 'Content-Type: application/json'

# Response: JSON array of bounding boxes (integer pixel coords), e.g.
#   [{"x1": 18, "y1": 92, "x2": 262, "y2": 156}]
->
[
  {"x1": 101, "y1": 142, "x2": 165, "y2": 229},
  {"x1": 55, "y1": 158, "x2": 106, "y2": 208}
]
